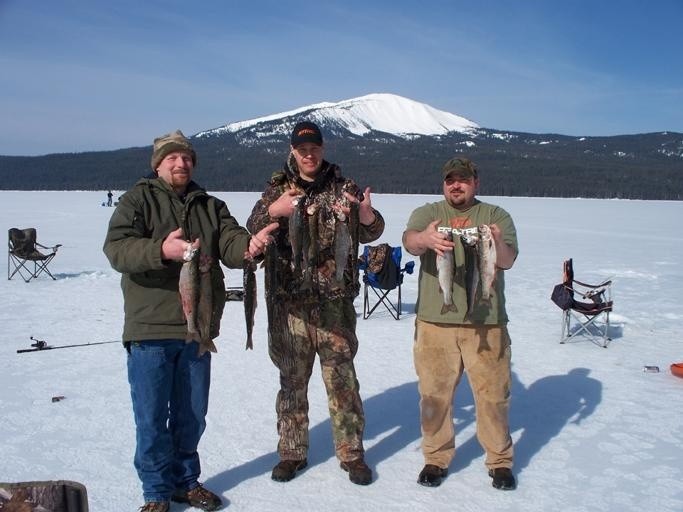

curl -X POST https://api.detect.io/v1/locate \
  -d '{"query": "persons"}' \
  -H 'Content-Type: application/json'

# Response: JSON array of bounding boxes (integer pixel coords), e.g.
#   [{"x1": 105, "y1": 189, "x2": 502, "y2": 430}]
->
[
  {"x1": 102, "y1": 126, "x2": 280, "y2": 512},
  {"x1": 402, "y1": 154, "x2": 520, "y2": 490},
  {"x1": 244, "y1": 121, "x2": 383, "y2": 485},
  {"x1": 106, "y1": 186, "x2": 113, "y2": 207}
]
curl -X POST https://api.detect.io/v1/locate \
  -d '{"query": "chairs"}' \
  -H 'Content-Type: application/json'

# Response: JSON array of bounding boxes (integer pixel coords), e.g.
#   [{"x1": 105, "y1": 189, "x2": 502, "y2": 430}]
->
[
  {"x1": 359, "y1": 245, "x2": 415, "y2": 320},
  {"x1": 7, "y1": 227, "x2": 62, "y2": 283},
  {"x1": 560, "y1": 258, "x2": 615, "y2": 348}
]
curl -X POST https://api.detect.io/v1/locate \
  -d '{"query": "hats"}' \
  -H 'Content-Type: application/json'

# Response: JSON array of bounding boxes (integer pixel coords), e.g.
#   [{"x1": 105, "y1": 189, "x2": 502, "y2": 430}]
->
[
  {"x1": 292, "y1": 122, "x2": 321, "y2": 146},
  {"x1": 441, "y1": 157, "x2": 477, "y2": 181},
  {"x1": 151, "y1": 131, "x2": 196, "y2": 172}
]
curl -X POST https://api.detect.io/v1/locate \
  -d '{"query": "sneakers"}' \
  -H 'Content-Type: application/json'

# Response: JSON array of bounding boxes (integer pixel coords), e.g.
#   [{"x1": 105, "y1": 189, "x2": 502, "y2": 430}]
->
[
  {"x1": 171, "y1": 488, "x2": 222, "y2": 511},
  {"x1": 489, "y1": 468, "x2": 515, "y2": 491},
  {"x1": 140, "y1": 503, "x2": 170, "y2": 512},
  {"x1": 341, "y1": 460, "x2": 371, "y2": 485},
  {"x1": 271, "y1": 457, "x2": 305, "y2": 481},
  {"x1": 419, "y1": 464, "x2": 447, "y2": 485}
]
